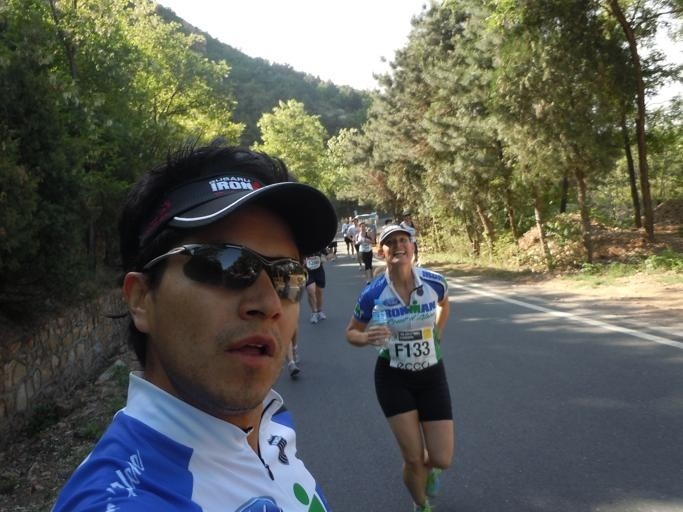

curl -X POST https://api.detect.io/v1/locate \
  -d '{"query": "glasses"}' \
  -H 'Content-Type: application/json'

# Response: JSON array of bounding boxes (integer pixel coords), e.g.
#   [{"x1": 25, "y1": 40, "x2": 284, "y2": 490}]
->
[{"x1": 145, "y1": 243, "x2": 310, "y2": 304}]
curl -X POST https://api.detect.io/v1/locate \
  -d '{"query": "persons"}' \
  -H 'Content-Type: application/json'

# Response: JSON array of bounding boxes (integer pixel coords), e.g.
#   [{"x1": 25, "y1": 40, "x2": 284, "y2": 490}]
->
[
  {"x1": 346, "y1": 225, "x2": 455, "y2": 512},
  {"x1": 51, "y1": 136, "x2": 338, "y2": 512},
  {"x1": 286, "y1": 212, "x2": 418, "y2": 377}
]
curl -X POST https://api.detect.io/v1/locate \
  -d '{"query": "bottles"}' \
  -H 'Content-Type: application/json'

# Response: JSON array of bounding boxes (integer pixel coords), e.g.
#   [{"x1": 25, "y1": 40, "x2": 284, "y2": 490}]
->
[{"x1": 372, "y1": 299, "x2": 387, "y2": 326}]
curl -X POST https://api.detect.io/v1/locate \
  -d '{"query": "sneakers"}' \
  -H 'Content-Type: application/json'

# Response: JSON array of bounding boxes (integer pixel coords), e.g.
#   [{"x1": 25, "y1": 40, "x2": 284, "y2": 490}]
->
[
  {"x1": 311, "y1": 310, "x2": 328, "y2": 324},
  {"x1": 351, "y1": 254, "x2": 373, "y2": 286},
  {"x1": 288, "y1": 345, "x2": 300, "y2": 376},
  {"x1": 413, "y1": 467, "x2": 441, "y2": 511}
]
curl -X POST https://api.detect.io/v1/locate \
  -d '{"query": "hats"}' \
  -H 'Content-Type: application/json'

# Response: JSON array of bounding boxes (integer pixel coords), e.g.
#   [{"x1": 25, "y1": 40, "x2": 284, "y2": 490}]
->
[
  {"x1": 128, "y1": 168, "x2": 338, "y2": 257},
  {"x1": 379, "y1": 225, "x2": 411, "y2": 247}
]
